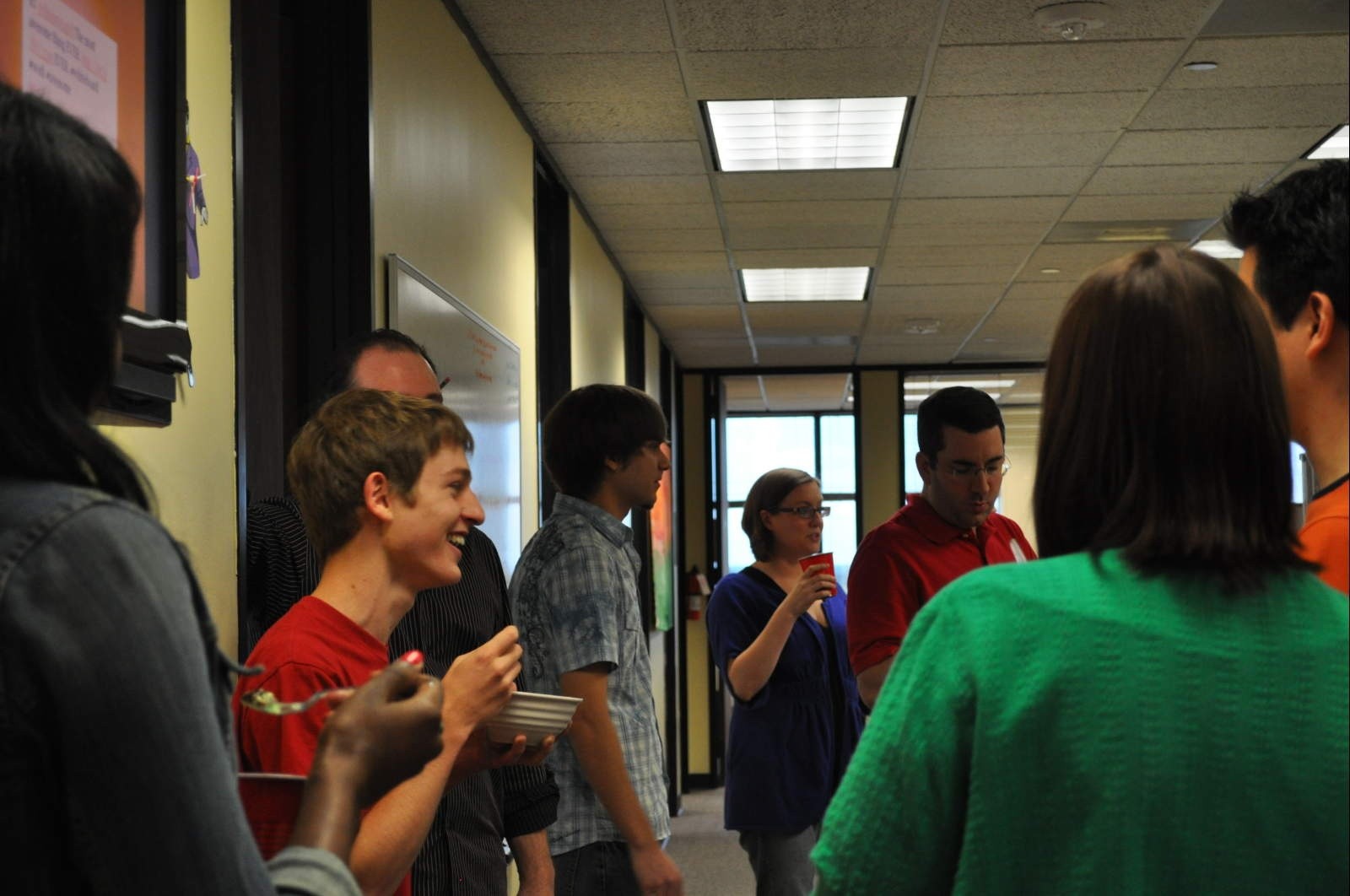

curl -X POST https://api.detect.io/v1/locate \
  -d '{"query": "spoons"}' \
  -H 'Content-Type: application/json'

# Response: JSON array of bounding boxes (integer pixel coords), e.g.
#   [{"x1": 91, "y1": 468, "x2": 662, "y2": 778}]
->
[{"x1": 241, "y1": 689, "x2": 357, "y2": 716}]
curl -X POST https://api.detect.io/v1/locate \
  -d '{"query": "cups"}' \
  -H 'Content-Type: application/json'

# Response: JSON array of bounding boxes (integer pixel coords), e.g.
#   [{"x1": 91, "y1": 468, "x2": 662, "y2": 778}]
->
[
  {"x1": 237, "y1": 774, "x2": 307, "y2": 863},
  {"x1": 799, "y1": 552, "x2": 838, "y2": 599}
]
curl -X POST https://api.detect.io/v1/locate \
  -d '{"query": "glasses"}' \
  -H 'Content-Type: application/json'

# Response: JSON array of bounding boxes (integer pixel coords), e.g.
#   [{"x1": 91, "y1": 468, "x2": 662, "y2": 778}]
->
[
  {"x1": 779, "y1": 504, "x2": 831, "y2": 519},
  {"x1": 936, "y1": 454, "x2": 1011, "y2": 484}
]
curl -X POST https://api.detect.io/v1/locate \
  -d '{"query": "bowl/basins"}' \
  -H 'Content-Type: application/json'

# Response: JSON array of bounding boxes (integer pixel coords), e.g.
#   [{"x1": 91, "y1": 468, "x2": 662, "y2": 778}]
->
[{"x1": 488, "y1": 692, "x2": 584, "y2": 747}]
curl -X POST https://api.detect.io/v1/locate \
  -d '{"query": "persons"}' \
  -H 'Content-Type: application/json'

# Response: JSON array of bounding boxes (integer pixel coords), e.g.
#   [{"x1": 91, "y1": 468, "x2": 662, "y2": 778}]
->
[
  {"x1": 847, "y1": 386, "x2": 1037, "y2": 713},
  {"x1": 238, "y1": 328, "x2": 559, "y2": 896},
  {"x1": 230, "y1": 387, "x2": 572, "y2": 896},
  {"x1": 507, "y1": 384, "x2": 684, "y2": 896},
  {"x1": 705, "y1": 468, "x2": 871, "y2": 896},
  {"x1": 806, "y1": 241, "x2": 1350, "y2": 896},
  {"x1": 0, "y1": 81, "x2": 444, "y2": 896},
  {"x1": 1221, "y1": 160, "x2": 1350, "y2": 595}
]
